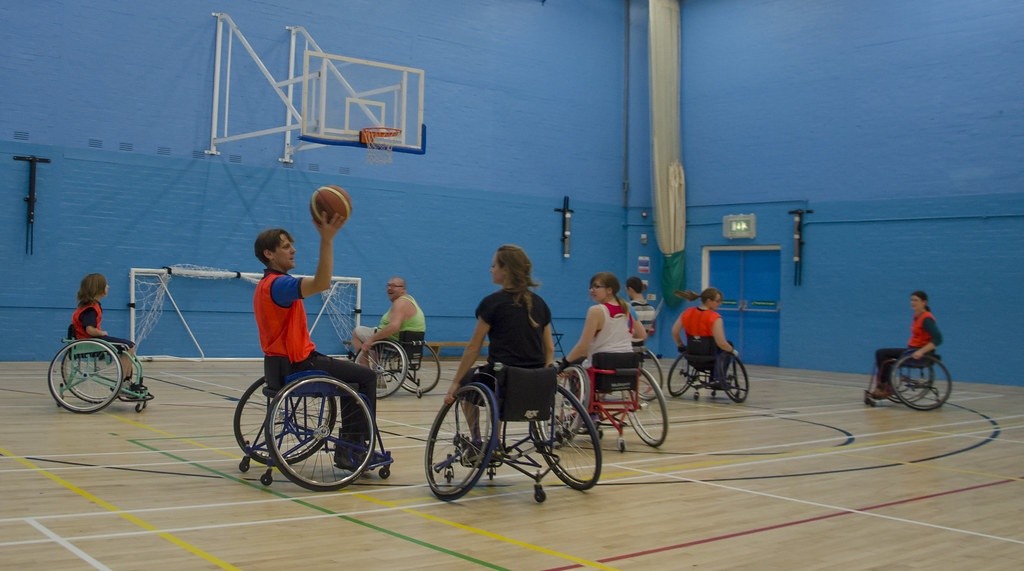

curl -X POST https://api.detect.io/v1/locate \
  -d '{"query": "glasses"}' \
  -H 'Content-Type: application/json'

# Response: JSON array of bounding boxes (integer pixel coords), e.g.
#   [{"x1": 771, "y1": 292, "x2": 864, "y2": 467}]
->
[
  {"x1": 386, "y1": 282, "x2": 403, "y2": 289},
  {"x1": 589, "y1": 282, "x2": 607, "y2": 289}
]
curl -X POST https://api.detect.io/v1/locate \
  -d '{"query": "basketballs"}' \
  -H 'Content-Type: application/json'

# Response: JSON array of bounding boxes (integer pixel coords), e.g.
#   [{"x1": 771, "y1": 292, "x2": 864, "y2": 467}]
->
[{"x1": 310, "y1": 185, "x2": 352, "y2": 224}]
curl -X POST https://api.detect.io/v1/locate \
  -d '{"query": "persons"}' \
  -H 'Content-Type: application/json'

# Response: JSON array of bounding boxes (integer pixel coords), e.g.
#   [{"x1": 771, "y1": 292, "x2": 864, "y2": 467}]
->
[
  {"x1": 71, "y1": 272, "x2": 146, "y2": 398},
  {"x1": 353, "y1": 277, "x2": 427, "y2": 389},
  {"x1": 556, "y1": 271, "x2": 647, "y2": 426},
  {"x1": 255, "y1": 212, "x2": 387, "y2": 470},
  {"x1": 444, "y1": 246, "x2": 554, "y2": 466},
  {"x1": 671, "y1": 287, "x2": 740, "y2": 388},
  {"x1": 624, "y1": 276, "x2": 656, "y2": 349},
  {"x1": 872, "y1": 291, "x2": 944, "y2": 400}
]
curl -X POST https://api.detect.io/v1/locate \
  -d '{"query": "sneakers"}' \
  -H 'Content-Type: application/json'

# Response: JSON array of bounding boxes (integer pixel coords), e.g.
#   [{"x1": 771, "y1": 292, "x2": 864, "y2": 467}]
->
[{"x1": 115, "y1": 378, "x2": 149, "y2": 399}]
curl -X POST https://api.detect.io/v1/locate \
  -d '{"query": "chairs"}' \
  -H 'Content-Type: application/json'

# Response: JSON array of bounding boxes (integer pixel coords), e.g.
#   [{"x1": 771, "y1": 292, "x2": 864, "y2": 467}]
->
[{"x1": 551, "y1": 320, "x2": 565, "y2": 357}]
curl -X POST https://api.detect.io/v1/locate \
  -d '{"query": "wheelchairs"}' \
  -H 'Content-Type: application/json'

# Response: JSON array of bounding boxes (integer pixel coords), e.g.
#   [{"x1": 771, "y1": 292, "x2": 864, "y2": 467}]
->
[
  {"x1": 48, "y1": 323, "x2": 155, "y2": 414},
  {"x1": 351, "y1": 335, "x2": 441, "y2": 400},
  {"x1": 233, "y1": 354, "x2": 394, "y2": 493},
  {"x1": 865, "y1": 346, "x2": 953, "y2": 412},
  {"x1": 424, "y1": 360, "x2": 603, "y2": 503},
  {"x1": 667, "y1": 335, "x2": 750, "y2": 404},
  {"x1": 528, "y1": 343, "x2": 671, "y2": 453}
]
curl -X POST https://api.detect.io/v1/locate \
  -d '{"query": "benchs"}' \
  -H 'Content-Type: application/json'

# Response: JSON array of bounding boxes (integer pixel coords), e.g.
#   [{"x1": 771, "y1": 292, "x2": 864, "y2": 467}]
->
[{"x1": 425, "y1": 342, "x2": 489, "y2": 361}]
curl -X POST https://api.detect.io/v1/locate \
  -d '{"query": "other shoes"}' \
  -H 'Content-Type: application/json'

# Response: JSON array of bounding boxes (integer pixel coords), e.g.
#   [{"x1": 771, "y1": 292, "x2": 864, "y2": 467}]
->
[
  {"x1": 709, "y1": 377, "x2": 730, "y2": 384},
  {"x1": 870, "y1": 381, "x2": 896, "y2": 398},
  {"x1": 376, "y1": 380, "x2": 388, "y2": 393},
  {"x1": 462, "y1": 440, "x2": 486, "y2": 463},
  {"x1": 483, "y1": 438, "x2": 505, "y2": 456},
  {"x1": 333, "y1": 441, "x2": 391, "y2": 468},
  {"x1": 582, "y1": 413, "x2": 601, "y2": 424}
]
